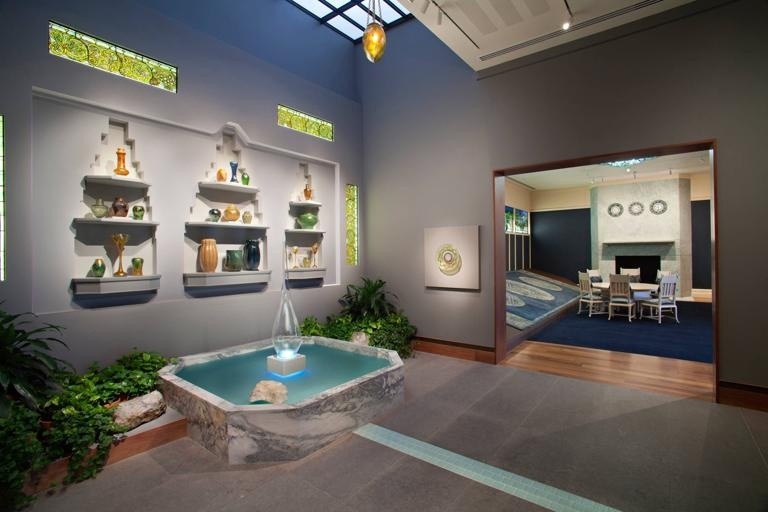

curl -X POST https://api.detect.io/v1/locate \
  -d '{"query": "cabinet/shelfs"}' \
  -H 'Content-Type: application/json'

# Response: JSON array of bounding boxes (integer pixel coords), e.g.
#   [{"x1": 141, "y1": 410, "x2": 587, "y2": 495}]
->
[
  {"x1": 184, "y1": 180, "x2": 272, "y2": 288},
  {"x1": 71, "y1": 174, "x2": 162, "y2": 295},
  {"x1": 284, "y1": 199, "x2": 327, "y2": 280}
]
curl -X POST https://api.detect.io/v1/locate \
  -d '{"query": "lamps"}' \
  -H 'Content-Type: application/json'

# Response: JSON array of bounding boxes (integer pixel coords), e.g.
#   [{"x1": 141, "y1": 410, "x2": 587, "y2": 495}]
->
[{"x1": 362, "y1": 0, "x2": 387, "y2": 64}]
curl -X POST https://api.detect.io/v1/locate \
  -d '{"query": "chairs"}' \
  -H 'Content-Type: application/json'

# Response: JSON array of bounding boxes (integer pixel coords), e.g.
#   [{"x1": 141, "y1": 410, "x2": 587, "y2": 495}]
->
[{"x1": 576, "y1": 266, "x2": 681, "y2": 324}]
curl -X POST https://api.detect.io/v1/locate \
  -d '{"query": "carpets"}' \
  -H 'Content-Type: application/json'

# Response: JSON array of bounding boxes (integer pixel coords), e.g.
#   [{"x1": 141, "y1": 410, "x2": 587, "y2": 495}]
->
[{"x1": 526, "y1": 300, "x2": 713, "y2": 364}]
